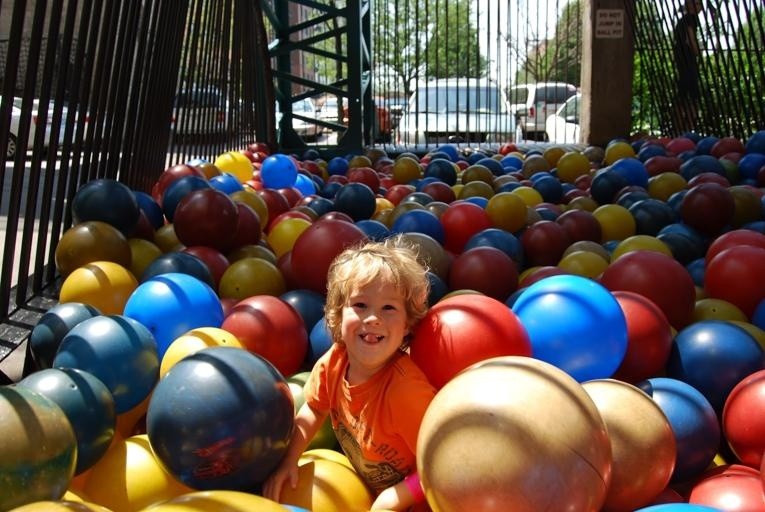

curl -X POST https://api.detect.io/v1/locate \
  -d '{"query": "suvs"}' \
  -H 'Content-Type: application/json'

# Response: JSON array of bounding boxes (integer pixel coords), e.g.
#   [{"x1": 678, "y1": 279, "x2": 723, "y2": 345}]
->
[{"x1": 506, "y1": 81, "x2": 577, "y2": 141}]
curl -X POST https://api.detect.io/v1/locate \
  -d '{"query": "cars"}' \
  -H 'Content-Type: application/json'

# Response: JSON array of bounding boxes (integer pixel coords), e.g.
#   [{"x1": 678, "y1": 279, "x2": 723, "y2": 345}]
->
[
  {"x1": 392, "y1": 77, "x2": 525, "y2": 150},
  {"x1": 172, "y1": 87, "x2": 235, "y2": 144},
  {"x1": 8, "y1": 97, "x2": 95, "y2": 156},
  {"x1": 544, "y1": 93, "x2": 583, "y2": 149},
  {"x1": 274, "y1": 97, "x2": 322, "y2": 140},
  {"x1": 318, "y1": 96, "x2": 409, "y2": 135}
]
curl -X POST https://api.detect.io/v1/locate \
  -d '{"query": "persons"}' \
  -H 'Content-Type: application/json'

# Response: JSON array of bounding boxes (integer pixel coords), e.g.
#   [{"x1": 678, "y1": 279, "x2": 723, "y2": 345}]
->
[
  {"x1": 670, "y1": 0, "x2": 703, "y2": 135},
  {"x1": 263, "y1": 235, "x2": 436, "y2": 512}
]
